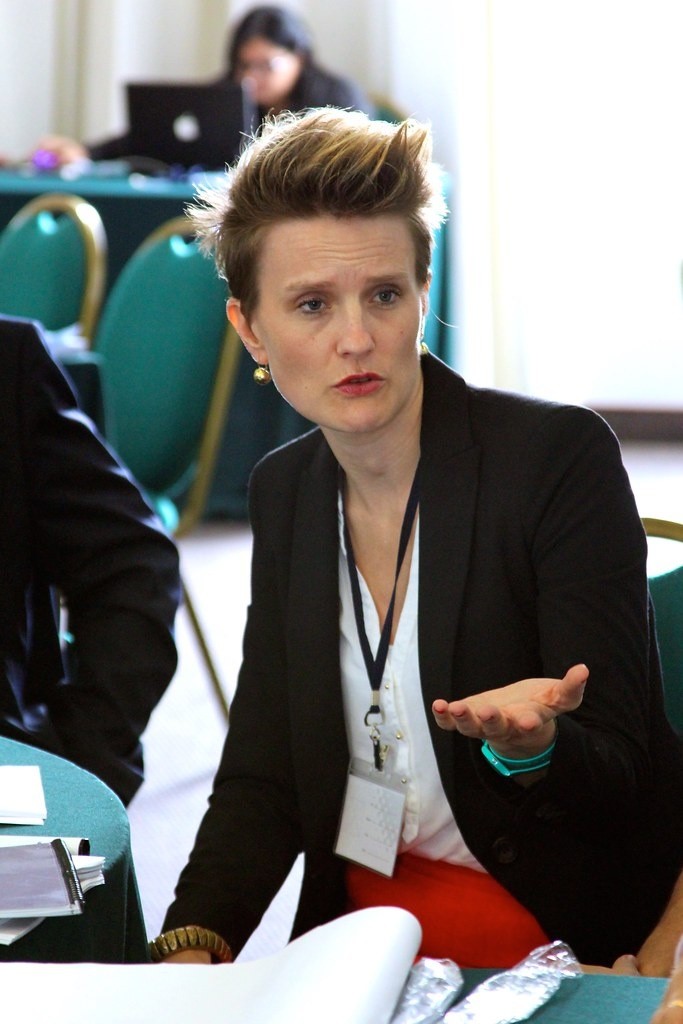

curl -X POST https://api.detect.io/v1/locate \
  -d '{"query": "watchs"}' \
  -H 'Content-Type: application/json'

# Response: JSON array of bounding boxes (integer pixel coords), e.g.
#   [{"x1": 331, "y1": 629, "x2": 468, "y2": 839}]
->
[{"x1": 480, "y1": 737, "x2": 555, "y2": 777}]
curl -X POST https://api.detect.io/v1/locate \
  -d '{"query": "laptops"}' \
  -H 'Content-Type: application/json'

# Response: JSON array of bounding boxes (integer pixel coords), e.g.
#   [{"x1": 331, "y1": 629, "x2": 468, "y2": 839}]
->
[{"x1": 124, "y1": 79, "x2": 255, "y2": 173}]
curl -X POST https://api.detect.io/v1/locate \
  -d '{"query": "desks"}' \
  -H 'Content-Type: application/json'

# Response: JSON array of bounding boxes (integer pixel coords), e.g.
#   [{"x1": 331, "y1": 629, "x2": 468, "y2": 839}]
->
[
  {"x1": 412, "y1": 967, "x2": 671, "y2": 1024},
  {"x1": 0, "y1": 735, "x2": 153, "y2": 962},
  {"x1": 0, "y1": 163, "x2": 237, "y2": 205}
]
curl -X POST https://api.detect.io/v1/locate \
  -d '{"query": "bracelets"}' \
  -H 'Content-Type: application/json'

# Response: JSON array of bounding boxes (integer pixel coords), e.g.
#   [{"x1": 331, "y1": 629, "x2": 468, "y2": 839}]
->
[
  {"x1": 667, "y1": 1000, "x2": 683, "y2": 1009},
  {"x1": 146, "y1": 925, "x2": 231, "y2": 964}
]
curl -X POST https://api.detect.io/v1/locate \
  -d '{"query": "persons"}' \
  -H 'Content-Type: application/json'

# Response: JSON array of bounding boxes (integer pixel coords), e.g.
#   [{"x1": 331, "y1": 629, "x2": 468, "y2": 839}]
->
[
  {"x1": 573, "y1": 867, "x2": 683, "y2": 1024},
  {"x1": 1, "y1": 314, "x2": 182, "y2": 806},
  {"x1": 144, "y1": 105, "x2": 683, "y2": 971},
  {"x1": 28, "y1": 6, "x2": 371, "y2": 171}
]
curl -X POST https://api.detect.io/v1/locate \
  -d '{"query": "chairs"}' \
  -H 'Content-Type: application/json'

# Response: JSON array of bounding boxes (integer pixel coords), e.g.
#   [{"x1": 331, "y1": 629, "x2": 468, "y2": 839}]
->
[{"x1": 0, "y1": 194, "x2": 243, "y2": 733}]
